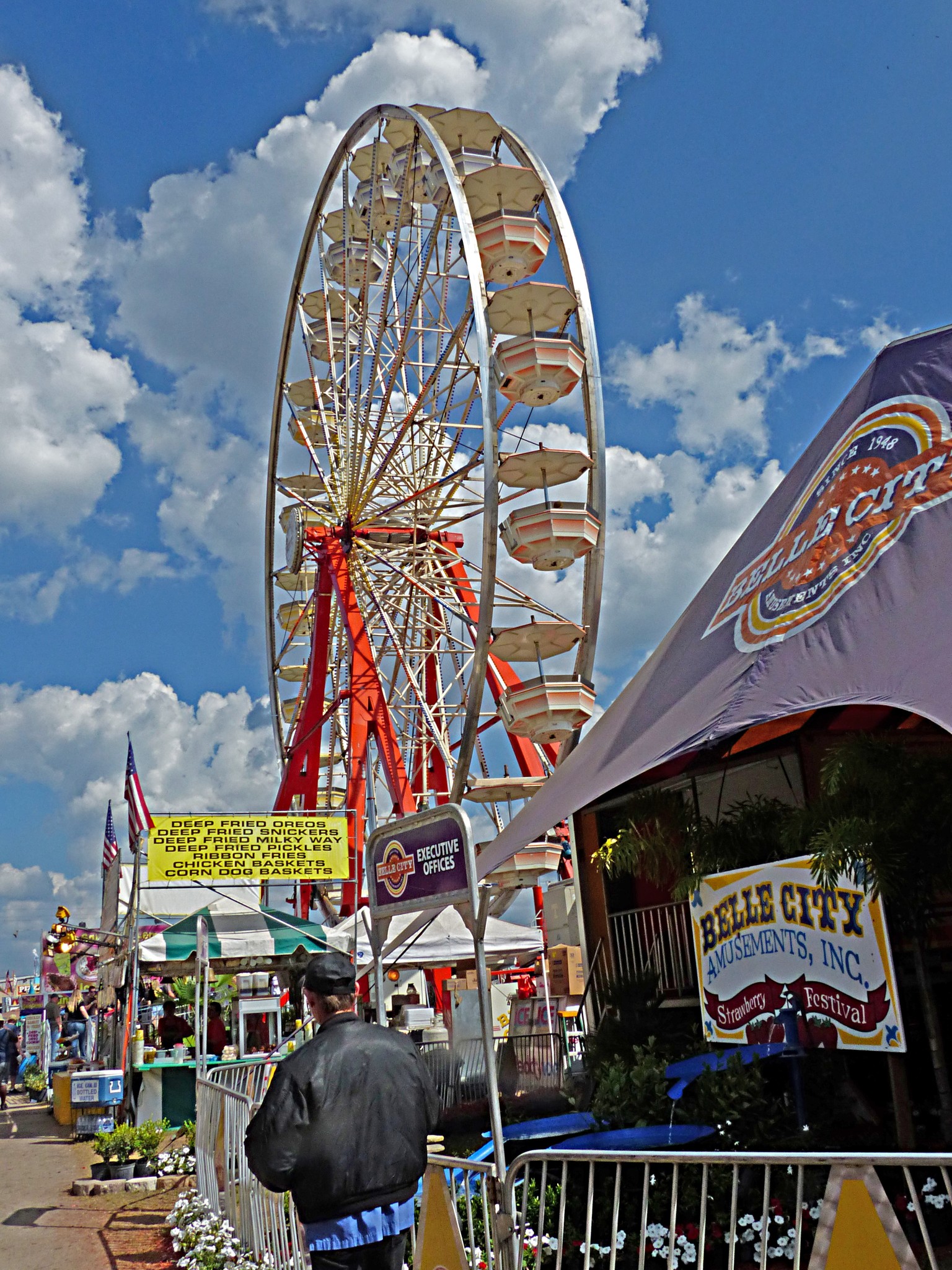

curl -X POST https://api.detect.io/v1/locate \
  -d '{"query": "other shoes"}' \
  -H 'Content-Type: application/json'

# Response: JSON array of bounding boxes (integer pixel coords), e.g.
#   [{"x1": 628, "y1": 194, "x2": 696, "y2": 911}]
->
[
  {"x1": 1, "y1": 1104, "x2": 8, "y2": 1110},
  {"x1": 9, "y1": 1088, "x2": 21, "y2": 1094}
]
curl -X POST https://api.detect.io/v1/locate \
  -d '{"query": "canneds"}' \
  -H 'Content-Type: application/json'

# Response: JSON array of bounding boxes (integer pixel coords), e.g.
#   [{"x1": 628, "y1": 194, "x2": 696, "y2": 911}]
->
[{"x1": 144, "y1": 1046, "x2": 154, "y2": 1064}]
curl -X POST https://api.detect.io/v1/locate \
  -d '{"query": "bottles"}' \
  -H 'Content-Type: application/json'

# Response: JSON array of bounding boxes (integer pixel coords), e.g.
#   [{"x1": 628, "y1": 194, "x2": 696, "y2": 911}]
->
[{"x1": 173, "y1": 1043, "x2": 184, "y2": 1064}]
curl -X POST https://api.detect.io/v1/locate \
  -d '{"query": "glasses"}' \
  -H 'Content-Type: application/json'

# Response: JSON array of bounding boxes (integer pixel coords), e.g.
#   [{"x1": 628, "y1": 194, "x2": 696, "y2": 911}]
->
[{"x1": 90, "y1": 991, "x2": 96, "y2": 992}]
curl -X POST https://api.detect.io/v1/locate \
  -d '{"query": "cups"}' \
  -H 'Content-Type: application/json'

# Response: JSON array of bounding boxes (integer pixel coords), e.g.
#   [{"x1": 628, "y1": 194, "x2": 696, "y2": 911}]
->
[
  {"x1": 295, "y1": 1015, "x2": 314, "y2": 1051},
  {"x1": 132, "y1": 1030, "x2": 145, "y2": 1066},
  {"x1": 287, "y1": 1041, "x2": 294, "y2": 1055}
]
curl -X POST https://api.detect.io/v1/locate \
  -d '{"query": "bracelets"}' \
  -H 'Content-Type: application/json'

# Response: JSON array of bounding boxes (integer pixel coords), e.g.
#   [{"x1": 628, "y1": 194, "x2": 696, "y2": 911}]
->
[{"x1": 59, "y1": 1025, "x2": 63, "y2": 1027}]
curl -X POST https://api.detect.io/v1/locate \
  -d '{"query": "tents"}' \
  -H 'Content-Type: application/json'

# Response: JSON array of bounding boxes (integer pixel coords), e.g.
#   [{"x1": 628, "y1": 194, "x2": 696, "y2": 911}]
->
[{"x1": 132, "y1": 893, "x2": 556, "y2": 1072}]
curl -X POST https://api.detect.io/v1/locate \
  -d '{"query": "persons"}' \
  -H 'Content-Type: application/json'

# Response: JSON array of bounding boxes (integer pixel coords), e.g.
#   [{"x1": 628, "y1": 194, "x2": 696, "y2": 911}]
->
[
  {"x1": 244, "y1": 952, "x2": 441, "y2": 1270},
  {"x1": 0, "y1": 984, "x2": 271, "y2": 1111}
]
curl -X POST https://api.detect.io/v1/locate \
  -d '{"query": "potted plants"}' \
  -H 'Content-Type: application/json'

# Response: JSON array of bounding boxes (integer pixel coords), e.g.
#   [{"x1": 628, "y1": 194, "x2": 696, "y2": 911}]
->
[
  {"x1": 24, "y1": 1073, "x2": 50, "y2": 1102},
  {"x1": 137, "y1": 1117, "x2": 170, "y2": 1177},
  {"x1": 107, "y1": 1121, "x2": 137, "y2": 1182},
  {"x1": 90, "y1": 1128, "x2": 115, "y2": 1181}
]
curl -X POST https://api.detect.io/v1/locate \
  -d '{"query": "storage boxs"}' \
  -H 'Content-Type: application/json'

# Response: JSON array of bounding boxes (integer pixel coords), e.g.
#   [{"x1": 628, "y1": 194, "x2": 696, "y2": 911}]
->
[
  {"x1": 457, "y1": 967, "x2": 492, "y2": 989},
  {"x1": 70, "y1": 1070, "x2": 124, "y2": 1107},
  {"x1": 441, "y1": 978, "x2": 467, "y2": 991},
  {"x1": 252, "y1": 971, "x2": 270, "y2": 996},
  {"x1": 546, "y1": 942, "x2": 586, "y2": 997},
  {"x1": 235, "y1": 972, "x2": 253, "y2": 997}
]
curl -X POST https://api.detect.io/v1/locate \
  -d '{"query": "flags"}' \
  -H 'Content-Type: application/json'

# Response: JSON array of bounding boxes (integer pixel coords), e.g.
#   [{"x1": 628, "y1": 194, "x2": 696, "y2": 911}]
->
[
  {"x1": 103, "y1": 804, "x2": 123, "y2": 894},
  {"x1": 5, "y1": 972, "x2": 11, "y2": 993},
  {"x1": 124, "y1": 740, "x2": 156, "y2": 850}
]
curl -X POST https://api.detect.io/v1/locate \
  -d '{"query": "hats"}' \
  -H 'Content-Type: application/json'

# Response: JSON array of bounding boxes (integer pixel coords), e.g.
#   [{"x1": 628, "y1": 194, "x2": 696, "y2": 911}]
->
[
  {"x1": 0, "y1": 1014, "x2": 5, "y2": 1021},
  {"x1": 7, "y1": 1014, "x2": 16, "y2": 1021},
  {"x1": 304, "y1": 953, "x2": 357, "y2": 994}
]
